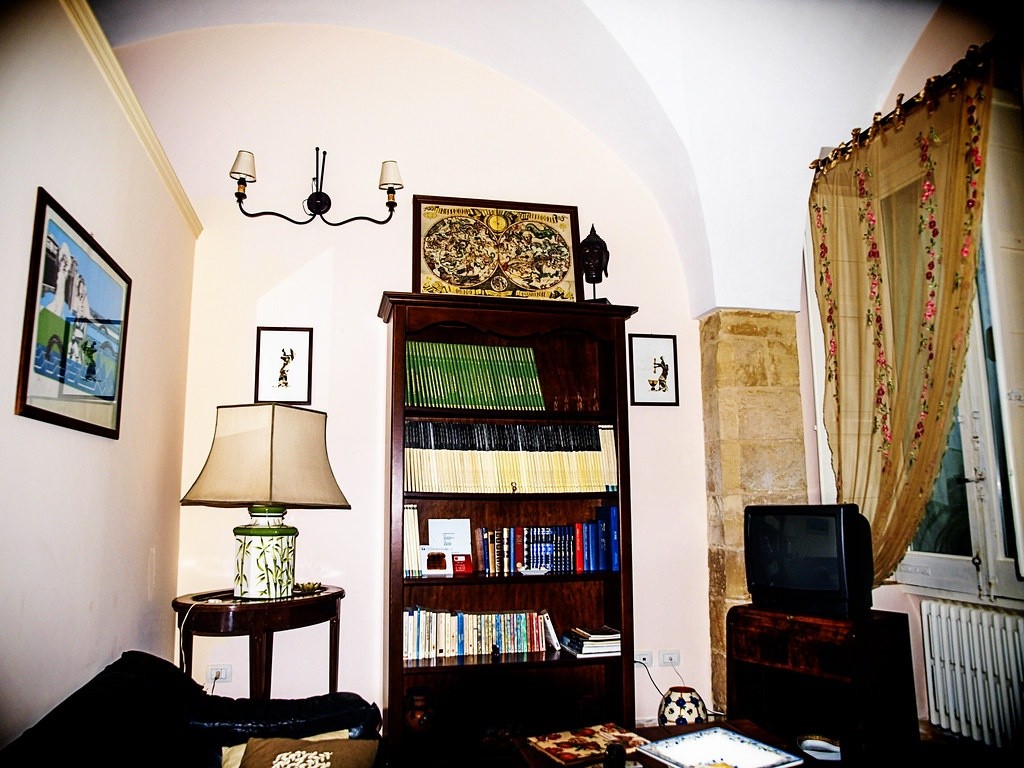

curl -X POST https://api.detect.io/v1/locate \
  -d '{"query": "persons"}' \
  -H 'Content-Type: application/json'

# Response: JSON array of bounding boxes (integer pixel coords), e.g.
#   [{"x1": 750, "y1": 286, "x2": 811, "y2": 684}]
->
[
  {"x1": 49, "y1": 255, "x2": 68, "y2": 317},
  {"x1": 72, "y1": 279, "x2": 89, "y2": 337}
]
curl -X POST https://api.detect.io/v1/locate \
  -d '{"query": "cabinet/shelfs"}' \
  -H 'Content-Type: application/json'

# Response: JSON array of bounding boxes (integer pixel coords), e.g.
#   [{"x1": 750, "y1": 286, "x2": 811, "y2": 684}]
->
[
  {"x1": 376, "y1": 291, "x2": 640, "y2": 768},
  {"x1": 723, "y1": 602, "x2": 923, "y2": 768}
]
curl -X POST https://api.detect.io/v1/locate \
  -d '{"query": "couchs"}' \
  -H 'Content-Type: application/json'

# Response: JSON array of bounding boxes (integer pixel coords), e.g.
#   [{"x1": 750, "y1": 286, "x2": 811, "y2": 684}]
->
[{"x1": 0, "y1": 649, "x2": 385, "y2": 768}]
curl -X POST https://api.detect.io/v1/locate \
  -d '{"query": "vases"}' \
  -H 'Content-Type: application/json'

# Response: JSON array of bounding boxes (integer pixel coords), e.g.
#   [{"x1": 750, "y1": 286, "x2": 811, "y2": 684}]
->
[{"x1": 658, "y1": 685, "x2": 708, "y2": 727}]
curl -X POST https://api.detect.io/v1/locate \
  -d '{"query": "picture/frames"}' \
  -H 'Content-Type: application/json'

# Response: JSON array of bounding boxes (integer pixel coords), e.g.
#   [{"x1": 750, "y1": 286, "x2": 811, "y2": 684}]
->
[
  {"x1": 253, "y1": 326, "x2": 314, "y2": 406},
  {"x1": 13, "y1": 186, "x2": 132, "y2": 441},
  {"x1": 628, "y1": 332, "x2": 681, "y2": 407},
  {"x1": 411, "y1": 196, "x2": 585, "y2": 304}
]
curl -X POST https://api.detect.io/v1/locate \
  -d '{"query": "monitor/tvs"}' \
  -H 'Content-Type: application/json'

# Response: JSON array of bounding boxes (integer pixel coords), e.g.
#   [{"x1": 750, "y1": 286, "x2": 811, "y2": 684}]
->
[{"x1": 744, "y1": 503, "x2": 873, "y2": 621}]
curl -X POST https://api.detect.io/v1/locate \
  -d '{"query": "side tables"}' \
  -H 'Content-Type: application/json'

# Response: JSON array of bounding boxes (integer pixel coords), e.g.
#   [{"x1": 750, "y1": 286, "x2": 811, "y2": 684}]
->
[{"x1": 167, "y1": 579, "x2": 346, "y2": 700}]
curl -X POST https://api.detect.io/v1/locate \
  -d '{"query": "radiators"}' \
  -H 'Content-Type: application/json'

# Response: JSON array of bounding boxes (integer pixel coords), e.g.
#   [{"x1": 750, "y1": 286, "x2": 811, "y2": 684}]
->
[{"x1": 920, "y1": 594, "x2": 1024, "y2": 756}]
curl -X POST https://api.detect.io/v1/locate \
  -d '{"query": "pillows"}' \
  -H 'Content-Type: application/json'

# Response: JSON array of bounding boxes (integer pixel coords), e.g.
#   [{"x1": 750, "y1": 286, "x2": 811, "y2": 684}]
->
[
  {"x1": 222, "y1": 729, "x2": 349, "y2": 768},
  {"x1": 238, "y1": 736, "x2": 379, "y2": 768}
]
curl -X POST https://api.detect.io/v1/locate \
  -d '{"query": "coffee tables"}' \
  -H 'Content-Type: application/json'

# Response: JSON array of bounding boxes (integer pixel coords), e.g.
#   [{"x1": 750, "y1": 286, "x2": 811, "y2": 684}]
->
[{"x1": 509, "y1": 717, "x2": 820, "y2": 768}]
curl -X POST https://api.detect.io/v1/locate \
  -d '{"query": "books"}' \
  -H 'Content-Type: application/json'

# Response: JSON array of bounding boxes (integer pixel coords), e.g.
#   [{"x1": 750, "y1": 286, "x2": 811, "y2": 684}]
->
[
  {"x1": 404, "y1": 420, "x2": 618, "y2": 494},
  {"x1": 405, "y1": 341, "x2": 545, "y2": 411},
  {"x1": 402, "y1": 504, "x2": 620, "y2": 580},
  {"x1": 403, "y1": 605, "x2": 622, "y2": 659}
]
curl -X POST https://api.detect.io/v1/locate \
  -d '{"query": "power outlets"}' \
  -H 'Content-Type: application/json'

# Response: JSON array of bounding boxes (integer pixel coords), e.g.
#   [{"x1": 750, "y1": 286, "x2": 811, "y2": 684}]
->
[{"x1": 206, "y1": 665, "x2": 232, "y2": 684}]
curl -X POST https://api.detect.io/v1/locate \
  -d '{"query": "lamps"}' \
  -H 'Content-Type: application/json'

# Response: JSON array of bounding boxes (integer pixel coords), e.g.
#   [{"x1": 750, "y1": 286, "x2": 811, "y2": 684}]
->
[
  {"x1": 181, "y1": 403, "x2": 355, "y2": 599},
  {"x1": 228, "y1": 146, "x2": 404, "y2": 225}
]
